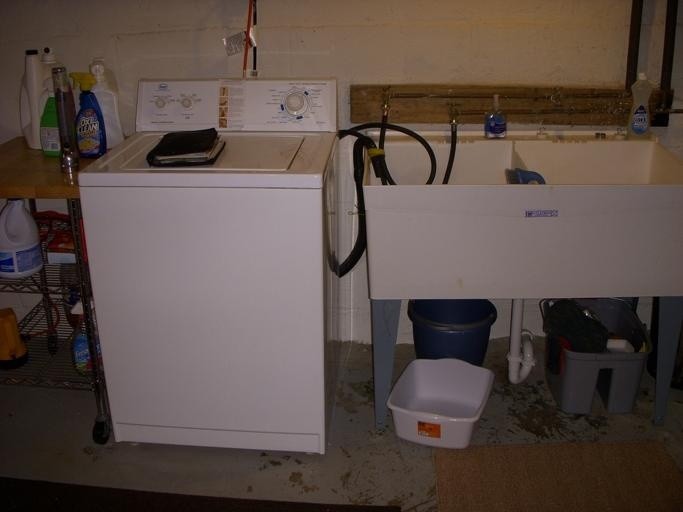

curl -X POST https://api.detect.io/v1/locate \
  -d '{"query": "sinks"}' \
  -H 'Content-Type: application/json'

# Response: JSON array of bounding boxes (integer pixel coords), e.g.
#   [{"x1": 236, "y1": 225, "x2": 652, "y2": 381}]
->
[
  {"x1": 514, "y1": 139, "x2": 683, "y2": 299},
  {"x1": 360, "y1": 137, "x2": 537, "y2": 300}
]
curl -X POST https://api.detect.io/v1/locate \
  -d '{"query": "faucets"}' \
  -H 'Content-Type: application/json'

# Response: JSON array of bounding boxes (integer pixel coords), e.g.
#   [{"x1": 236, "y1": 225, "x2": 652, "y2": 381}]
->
[
  {"x1": 380, "y1": 96, "x2": 390, "y2": 117},
  {"x1": 448, "y1": 108, "x2": 459, "y2": 125}
]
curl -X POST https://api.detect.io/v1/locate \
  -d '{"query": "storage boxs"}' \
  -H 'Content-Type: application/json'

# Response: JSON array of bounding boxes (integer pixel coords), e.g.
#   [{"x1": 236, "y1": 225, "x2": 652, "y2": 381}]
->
[
  {"x1": 388, "y1": 359, "x2": 493, "y2": 450},
  {"x1": 539, "y1": 298, "x2": 652, "y2": 413}
]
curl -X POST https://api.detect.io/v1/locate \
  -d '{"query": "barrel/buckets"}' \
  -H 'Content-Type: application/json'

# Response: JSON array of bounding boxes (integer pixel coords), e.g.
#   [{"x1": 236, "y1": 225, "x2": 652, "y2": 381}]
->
[
  {"x1": 408, "y1": 300, "x2": 497, "y2": 366},
  {"x1": 0, "y1": 199, "x2": 44, "y2": 279}
]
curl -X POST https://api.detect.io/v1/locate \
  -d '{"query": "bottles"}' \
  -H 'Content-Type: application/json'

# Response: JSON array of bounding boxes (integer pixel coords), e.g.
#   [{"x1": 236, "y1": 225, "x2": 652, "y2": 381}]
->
[
  {"x1": 627, "y1": 72, "x2": 653, "y2": 139},
  {"x1": 50, "y1": 66, "x2": 78, "y2": 174}
]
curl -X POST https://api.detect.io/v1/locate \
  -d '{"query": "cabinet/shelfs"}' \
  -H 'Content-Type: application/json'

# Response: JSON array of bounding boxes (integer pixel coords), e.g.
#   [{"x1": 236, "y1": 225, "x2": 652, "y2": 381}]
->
[{"x1": 0, "y1": 136, "x2": 113, "y2": 445}]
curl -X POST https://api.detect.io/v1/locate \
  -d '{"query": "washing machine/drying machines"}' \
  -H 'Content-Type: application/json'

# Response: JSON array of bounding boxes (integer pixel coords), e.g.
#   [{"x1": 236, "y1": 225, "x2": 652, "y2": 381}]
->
[{"x1": 78, "y1": 79, "x2": 341, "y2": 454}]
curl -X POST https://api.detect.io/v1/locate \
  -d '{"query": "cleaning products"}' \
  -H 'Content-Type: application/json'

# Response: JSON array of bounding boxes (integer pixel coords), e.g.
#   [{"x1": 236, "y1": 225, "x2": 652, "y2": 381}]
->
[
  {"x1": 19, "y1": 49, "x2": 123, "y2": 158},
  {"x1": 70, "y1": 299, "x2": 104, "y2": 377}
]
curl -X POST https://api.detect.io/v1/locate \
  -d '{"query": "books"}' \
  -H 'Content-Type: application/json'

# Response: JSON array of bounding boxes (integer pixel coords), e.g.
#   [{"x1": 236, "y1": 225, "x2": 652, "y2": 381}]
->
[{"x1": 145, "y1": 127, "x2": 226, "y2": 167}]
